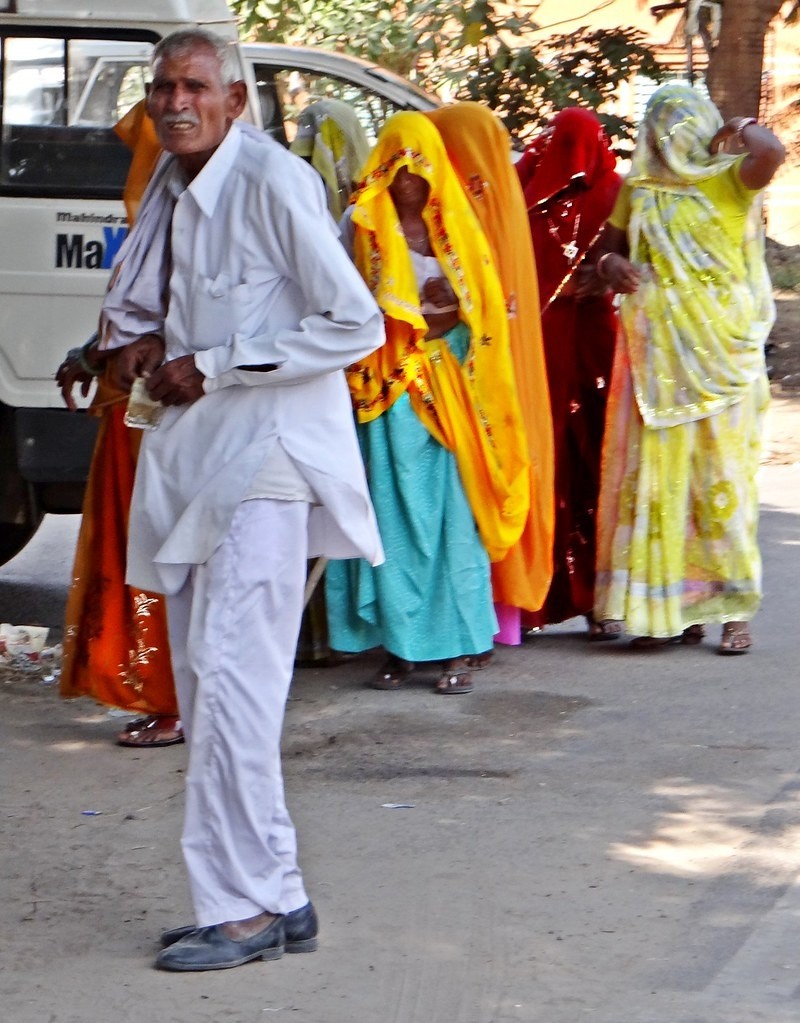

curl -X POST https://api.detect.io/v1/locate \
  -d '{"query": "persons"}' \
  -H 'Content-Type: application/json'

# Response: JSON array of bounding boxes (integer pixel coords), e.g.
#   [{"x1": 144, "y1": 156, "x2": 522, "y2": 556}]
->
[{"x1": 55, "y1": 28, "x2": 786, "y2": 971}]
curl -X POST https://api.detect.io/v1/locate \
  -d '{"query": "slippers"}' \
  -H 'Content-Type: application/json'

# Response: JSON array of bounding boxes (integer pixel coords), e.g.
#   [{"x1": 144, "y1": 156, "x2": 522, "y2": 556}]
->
[
  {"x1": 722, "y1": 628, "x2": 752, "y2": 648},
  {"x1": 438, "y1": 669, "x2": 474, "y2": 692},
  {"x1": 593, "y1": 622, "x2": 621, "y2": 640},
  {"x1": 683, "y1": 631, "x2": 703, "y2": 644},
  {"x1": 468, "y1": 649, "x2": 495, "y2": 670},
  {"x1": 118, "y1": 715, "x2": 186, "y2": 748},
  {"x1": 369, "y1": 669, "x2": 417, "y2": 689}
]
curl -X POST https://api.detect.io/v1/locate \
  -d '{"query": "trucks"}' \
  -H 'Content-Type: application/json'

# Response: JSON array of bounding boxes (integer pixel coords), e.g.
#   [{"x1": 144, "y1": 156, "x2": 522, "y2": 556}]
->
[{"x1": 0, "y1": 1, "x2": 450, "y2": 569}]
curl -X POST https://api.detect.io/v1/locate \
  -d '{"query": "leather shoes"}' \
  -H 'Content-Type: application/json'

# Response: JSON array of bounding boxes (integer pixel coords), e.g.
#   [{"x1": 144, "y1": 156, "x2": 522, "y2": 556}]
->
[
  {"x1": 156, "y1": 916, "x2": 286, "y2": 970},
  {"x1": 160, "y1": 900, "x2": 319, "y2": 953}
]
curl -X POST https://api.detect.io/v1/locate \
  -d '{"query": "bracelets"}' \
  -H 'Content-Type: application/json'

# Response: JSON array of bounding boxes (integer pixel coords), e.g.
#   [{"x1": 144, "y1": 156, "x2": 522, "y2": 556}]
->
[
  {"x1": 735, "y1": 116, "x2": 758, "y2": 148},
  {"x1": 597, "y1": 252, "x2": 614, "y2": 279},
  {"x1": 67, "y1": 349, "x2": 104, "y2": 375}
]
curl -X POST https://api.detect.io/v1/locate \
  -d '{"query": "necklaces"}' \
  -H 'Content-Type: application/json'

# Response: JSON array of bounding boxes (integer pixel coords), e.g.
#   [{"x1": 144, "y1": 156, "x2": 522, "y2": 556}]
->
[{"x1": 405, "y1": 236, "x2": 428, "y2": 243}]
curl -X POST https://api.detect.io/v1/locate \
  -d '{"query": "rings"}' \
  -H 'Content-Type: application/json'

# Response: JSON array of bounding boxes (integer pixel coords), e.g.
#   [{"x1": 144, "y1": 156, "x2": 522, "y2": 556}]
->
[{"x1": 62, "y1": 367, "x2": 70, "y2": 373}]
